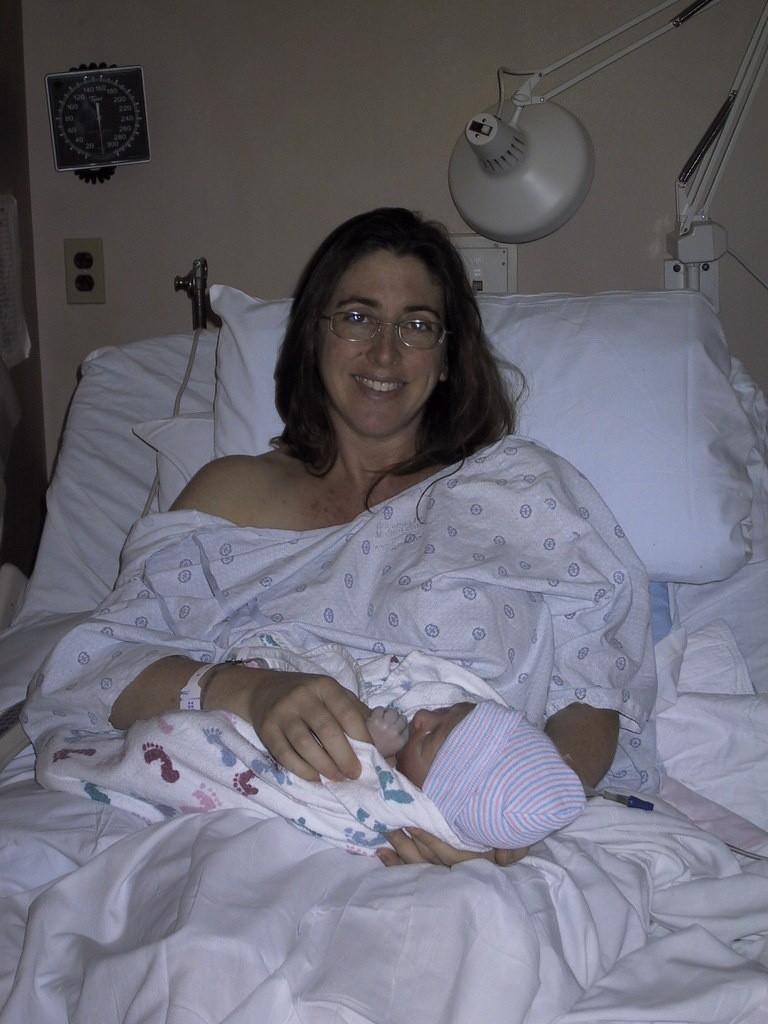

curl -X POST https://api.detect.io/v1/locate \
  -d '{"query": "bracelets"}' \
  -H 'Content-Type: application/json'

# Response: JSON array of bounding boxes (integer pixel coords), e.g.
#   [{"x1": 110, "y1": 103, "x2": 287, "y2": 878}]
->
[{"x1": 177, "y1": 661, "x2": 227, "y2": 712}]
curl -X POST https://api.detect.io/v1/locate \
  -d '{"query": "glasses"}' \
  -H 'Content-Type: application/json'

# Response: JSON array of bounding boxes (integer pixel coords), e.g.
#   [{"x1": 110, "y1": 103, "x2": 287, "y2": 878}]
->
[{"x1": 318, "y1": 310, "x2": 452, "y2": 349}]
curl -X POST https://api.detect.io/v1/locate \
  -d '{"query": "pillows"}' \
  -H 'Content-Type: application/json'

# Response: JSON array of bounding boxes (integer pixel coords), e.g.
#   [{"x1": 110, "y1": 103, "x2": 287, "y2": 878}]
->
[
  {"x1": 133, "y1": 410, "x2": 690, "y2": 715},
  {"x1": 208, "y1": 284, "x2": 760, "y2": 585}
]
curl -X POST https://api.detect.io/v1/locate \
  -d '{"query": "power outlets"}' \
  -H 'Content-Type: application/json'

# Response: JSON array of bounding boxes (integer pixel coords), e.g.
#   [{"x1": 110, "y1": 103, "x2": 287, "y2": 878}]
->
[{"x1": 64, "y1": 238, "x2": 105, "y2": 304}]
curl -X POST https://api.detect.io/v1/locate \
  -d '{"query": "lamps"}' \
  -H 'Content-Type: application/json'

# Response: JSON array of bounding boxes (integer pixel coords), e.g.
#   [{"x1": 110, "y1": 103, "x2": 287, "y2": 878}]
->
[{"x1": 447, "y1": 0, "x2": 768, "y2": 315}]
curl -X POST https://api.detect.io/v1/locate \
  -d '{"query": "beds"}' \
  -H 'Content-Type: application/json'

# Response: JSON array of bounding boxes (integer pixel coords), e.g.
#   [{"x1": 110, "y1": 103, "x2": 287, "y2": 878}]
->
[{"x1": 0, "y1": 327, "x2": 768, "y2": 1024}]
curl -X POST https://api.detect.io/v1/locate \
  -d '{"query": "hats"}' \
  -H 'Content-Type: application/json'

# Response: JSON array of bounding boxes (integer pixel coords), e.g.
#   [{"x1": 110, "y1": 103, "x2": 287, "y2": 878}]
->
[{"x1": 421, "y1": 698, "x2": 588, "y2": 849}]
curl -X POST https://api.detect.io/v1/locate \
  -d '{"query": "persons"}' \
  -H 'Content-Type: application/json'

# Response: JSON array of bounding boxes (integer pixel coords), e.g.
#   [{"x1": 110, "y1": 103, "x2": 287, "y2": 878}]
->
[{"x1": 21, "y1": 210, "x2": 653, "y2": 867}]
